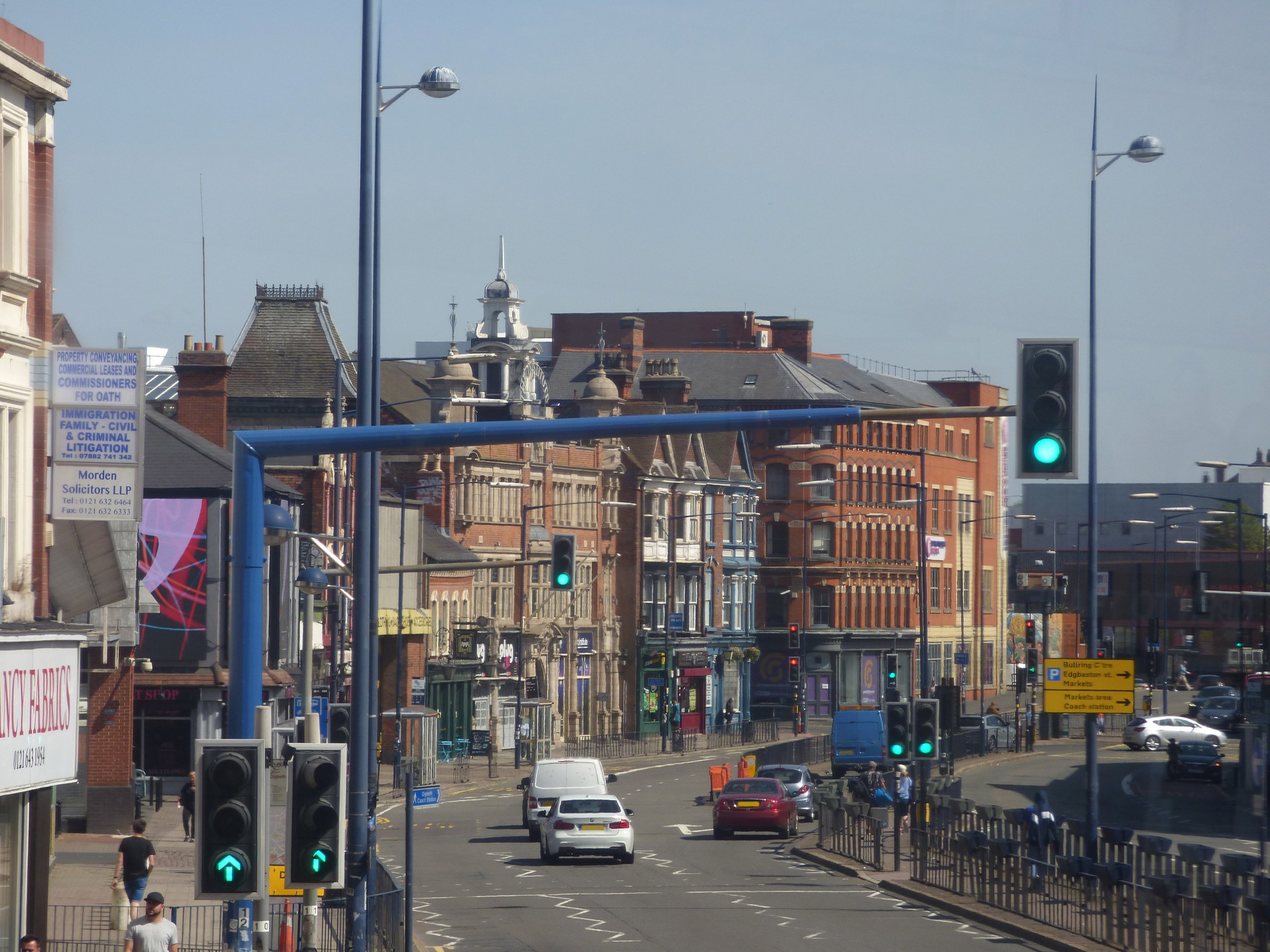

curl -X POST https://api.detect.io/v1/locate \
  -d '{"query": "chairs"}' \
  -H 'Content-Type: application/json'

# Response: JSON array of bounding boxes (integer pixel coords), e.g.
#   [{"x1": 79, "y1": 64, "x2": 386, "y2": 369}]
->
[{"x1": 438, "y1": 738, "x2": 471, "y2": 764}]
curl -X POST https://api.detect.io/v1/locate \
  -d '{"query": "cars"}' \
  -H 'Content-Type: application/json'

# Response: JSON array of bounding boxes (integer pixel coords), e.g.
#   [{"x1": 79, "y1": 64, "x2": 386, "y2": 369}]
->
[
  {"x1": 712, "y1": 670, "x2": 1270, "y2": 841},
  {"x1": 516, "y1": 784, "x2": 530, "y2": 829},
  {"x1": 537, "y1": 793, "x2": 635, "y2": 864}
]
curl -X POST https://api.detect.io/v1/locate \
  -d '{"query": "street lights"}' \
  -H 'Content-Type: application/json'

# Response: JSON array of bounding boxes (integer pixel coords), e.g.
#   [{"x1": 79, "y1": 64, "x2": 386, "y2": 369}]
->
[
  {"x1": 1083, "y1": 73, "x2": 1166, "y2": 895},
  {"x1": 774, "y1": 442, "x2": 1270, "y2": 851},
  {"x1": 342, "y1": 2, "x2": 466, "y2": 952},
  {"x1": 393, "y1": 479, "x2": 638, "y2": 790}
]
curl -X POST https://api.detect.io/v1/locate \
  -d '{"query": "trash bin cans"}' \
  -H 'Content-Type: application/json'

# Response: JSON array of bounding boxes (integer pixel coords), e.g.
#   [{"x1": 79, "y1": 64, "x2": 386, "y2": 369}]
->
[
  {"x1": 741, "y1": 719, "x2": 755, "y2": 742},
  {"x1": 673, "y1": 727, "x2": 683, "y2": 752}
]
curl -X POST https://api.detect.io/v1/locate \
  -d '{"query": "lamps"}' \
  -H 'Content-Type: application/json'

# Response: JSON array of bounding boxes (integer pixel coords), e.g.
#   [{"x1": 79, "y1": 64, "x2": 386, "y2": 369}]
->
[
  {"x1": 495, "y1": 542, "x2": 500, "y2": 546},
  {"x1": 479, "y1": 649, "x2": 497, "y2": 677},
  {"x1": 438, "y1": 646, "x2": 457, "y2": 680},
  {"x1": 576, "y1": 661, "x2": 584, "y2": 667},
  {"x1": 722, "y1": 645, "x2": 734, "y2": 664}
]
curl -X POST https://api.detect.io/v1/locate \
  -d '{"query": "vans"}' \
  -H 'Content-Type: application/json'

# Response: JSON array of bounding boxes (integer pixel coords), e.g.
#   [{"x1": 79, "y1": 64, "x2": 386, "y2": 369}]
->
[{"x1": 521, "y1": 756, "x2": 618, "y2": 842}]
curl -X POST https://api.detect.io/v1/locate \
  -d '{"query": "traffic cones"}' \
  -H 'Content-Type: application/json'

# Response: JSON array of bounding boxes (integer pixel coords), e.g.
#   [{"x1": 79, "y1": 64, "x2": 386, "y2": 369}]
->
[
  {"x1": 796, "y1": 711, "x2": 801, "y2": 733},
  {"x1": 278, "y1": 898, "x2": 296, "y2": 952}
]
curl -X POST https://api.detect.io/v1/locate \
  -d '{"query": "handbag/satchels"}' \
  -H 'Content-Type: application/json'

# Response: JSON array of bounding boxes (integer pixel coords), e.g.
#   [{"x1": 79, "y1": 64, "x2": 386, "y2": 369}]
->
[
  {"x1": 873, "y1": 788, "x2": 893, "y2": 805},
  {"x1": 179, "y1": 784, "x2": 186, "y2": 805}
]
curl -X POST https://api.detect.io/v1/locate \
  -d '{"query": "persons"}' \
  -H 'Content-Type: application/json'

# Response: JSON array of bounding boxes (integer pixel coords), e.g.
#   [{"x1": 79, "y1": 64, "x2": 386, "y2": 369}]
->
[
  {"x1": 725, "y1": 697, "x2": 736, "y2": 735},
  {"x1": 986, "y1": 701, "x2": 1002, "y2": 717},
  {"x1": 520, "y1": 716, "x2": 529, "y2": 759},
  {"x1": 1026, "y1": 790, "x2": 1059, "y2": 892},
  {"x1": 1173, "y1": 661, "x2": 1194, "y2": 692},
  {"x1": 124, "y1": 892, "x2": 179, "y2": 952},
  {"x1": 928, "y1": 680, "x2": 938, "y2": 699},
  {"x1": 179, "y1": 771, "x2": 195, "y2": 843},
  {"x1": 110, "y1": 818, "x2": 156, "y2": 920},
  {"x1": 859, "y1": 761, "x2": 886, "y2": 835},
  {"x1": 891, "y1": 764, "x2": 912, "y2": 834},
  {"x1": 1094, "y1": 713, "x2": 1107, "y2": 736},
  {"x1": 669, "y1": 697, "x2": 681, "y2": 744},
  {"x1": 19, "y1": 935, "x2": 41, "y2": 952}
]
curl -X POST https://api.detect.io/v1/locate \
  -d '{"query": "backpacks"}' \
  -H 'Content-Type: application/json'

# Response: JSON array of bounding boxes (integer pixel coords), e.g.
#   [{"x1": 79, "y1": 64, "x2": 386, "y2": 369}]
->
[{"x1": 848, "y1": 772, "x2": 869, "y2": 798}]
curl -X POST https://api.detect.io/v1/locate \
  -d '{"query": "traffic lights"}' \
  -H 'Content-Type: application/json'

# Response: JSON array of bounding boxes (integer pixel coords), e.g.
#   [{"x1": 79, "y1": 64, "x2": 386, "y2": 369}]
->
[
  {"x1": 789, "y1": 623, "x2": 800, "y2": 649},
  {"x1": 789, "y1": 657, "x2": 800, "y2": 682},
  {"x1": 550, "y1": 532, "x2": 576, "y2": 593},
  {"x1": 327, "y1": 703, "x2": 351, "y2": 767},
  {"x1": 193, "y1": 738, "x2": 265, "y2": 903},
  {"x1": 1025, "y1": 618, "x2": 1036, "y2": 649},
  {"x1": 1026, "y1": 649, "x2": 1039, "y2": 683},
  {"x1": 1017, "y1": 337, "x2": 1080, "y2": 481},
  {"x1": 911, "y1": 698, "x2": 939, "y2": 761},
  {"x1": 885, "y1": 701, "x2": 910, "y2": 762},
  {"x1": 286, "y1": 742, "x2": 345, "y2": 890},
  {"x1": 886, "y1": 653, "x2": 898, "y2": 689},
  {"x1": 1097, "y1": 648, "x2": 1107, "y2": 659}
]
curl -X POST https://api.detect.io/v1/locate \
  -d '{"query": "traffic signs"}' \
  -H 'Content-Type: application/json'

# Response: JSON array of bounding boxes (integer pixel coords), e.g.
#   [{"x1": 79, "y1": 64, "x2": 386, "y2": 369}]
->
[
  {"x1": 1043, "y1": 691, "x2": 1134, "y2": 714},
  {"x1": 1043, "y1": 658, "x2": 1135, "y2": 691},
  {"x1": 412, "y1": 787, "x2": 441, "y2": 806}
]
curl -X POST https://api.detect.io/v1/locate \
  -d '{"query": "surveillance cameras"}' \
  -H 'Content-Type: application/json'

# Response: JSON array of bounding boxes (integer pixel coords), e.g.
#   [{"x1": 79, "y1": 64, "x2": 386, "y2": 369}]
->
[{"x1": 141, "y1": 662, "x2": 152, "y2": 674}]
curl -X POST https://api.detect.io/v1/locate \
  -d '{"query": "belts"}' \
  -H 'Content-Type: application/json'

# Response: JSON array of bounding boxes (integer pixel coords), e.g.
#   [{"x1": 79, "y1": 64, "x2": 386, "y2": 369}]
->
[{"x1": 1180, "y1": 674, "x2": 1185, "y2": 675}]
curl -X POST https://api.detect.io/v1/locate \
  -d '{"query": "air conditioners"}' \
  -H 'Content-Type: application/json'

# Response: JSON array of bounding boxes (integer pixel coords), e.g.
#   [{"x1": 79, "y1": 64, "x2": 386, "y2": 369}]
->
[
  {"x1": 1016, "y1": 573, "x2": 1028, "y2": 586},
  {"x1": 1042, "y1": 576, "x2": 1051, "y2": 586}
]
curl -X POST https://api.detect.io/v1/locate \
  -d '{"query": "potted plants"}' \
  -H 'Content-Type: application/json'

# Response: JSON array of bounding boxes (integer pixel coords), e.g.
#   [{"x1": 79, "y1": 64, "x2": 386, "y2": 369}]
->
[
  {"x1": 744, "y1": 647, "x2": 760, "y2": 663},
  {"x1": 730, "y1": 646, "x2": 745, "y2": 663}
]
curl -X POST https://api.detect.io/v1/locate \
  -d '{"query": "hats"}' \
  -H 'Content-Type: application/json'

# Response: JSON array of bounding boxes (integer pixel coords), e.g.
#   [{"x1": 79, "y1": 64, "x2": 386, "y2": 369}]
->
[
  {"x1": 897, "y1": 764, "x2": 906, "y2": 773},
  {"x1": 869, "y1": 760, "x2": 877, "y2": 767},
  {"x1": 143, "y1": 892, "x2": 164, "y2": 904}
]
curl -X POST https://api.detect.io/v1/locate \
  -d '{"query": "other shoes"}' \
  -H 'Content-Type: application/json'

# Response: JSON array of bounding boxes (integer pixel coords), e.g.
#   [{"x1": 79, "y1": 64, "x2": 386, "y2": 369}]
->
[
  {"x1": 865, "y1": 828, "x2": 871, "y2": 835},
  {"x1": 900, "y1": 830, "x2": 902, "y2": 834},
  {"x1": 184, "y1": 835, "x2": 189, "y2": 841},
  {"x1": 1188, "y1": 688, "x2": 1193, "y2": 691},
  {"x1": 191, "y1": 839, "x2": 194, "y2": 842},
  {"x1": 1173, "y1": 689, "x2": 1177, "y2": 691},
  {"x1": 903, "y1": 822, "x2": 909, "y2": 833}
]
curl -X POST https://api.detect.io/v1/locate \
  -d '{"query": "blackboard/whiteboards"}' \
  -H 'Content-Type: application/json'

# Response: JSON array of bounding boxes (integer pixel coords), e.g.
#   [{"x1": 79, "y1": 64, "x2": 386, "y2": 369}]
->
[{"x1": 467, "y1": 730, "x2": 491, "y2": 756}]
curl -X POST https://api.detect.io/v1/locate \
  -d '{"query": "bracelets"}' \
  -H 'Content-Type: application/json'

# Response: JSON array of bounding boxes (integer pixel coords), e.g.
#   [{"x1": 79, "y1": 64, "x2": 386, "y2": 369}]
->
[{"x1": 114, "y1": 876, "x2": 119, "y2": 879}]
